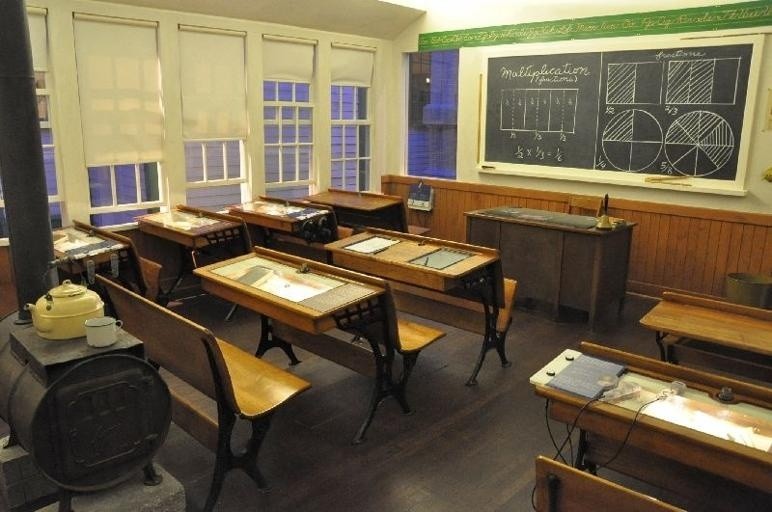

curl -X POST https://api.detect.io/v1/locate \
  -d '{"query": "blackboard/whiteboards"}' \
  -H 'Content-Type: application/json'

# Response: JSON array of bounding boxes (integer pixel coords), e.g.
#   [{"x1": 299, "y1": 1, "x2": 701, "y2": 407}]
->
[{"x1": 476, "y1": 33, "x2": 765, "y2": 198}]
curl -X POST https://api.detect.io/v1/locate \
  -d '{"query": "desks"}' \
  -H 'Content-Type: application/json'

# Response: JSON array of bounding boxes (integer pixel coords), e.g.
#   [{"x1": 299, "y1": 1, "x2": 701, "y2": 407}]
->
[
  {"x1": 323, "y1": 226, "x2": 512, "y2": 386},
  {"x1": 530, "y1": 342, "x2": 772, "y2": 499},
  {"x1": 637, "y1": 290, "x2": 772, "y2": 389},
  {"x1": 229, "y1": 195, "x2": 339, "y2": 265},
  {"x1": 132, "y1": 205, "x2": 253, "y2": 321},
  {"x1": 305, "y1": 188, "x2": 409, "y2": 233},
  {"x1": 53, "y1": 219, "x2": 147, "y2": 297},
  {"x1": 192, "y1": 246, "x2": 400, "y2": 444}
]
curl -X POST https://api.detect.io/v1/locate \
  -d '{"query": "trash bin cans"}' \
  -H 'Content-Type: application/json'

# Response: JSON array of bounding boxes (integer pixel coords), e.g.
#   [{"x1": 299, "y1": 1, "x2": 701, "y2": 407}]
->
[{"x1": 725, "y1": 272, "x2": 772, "y2": 310}]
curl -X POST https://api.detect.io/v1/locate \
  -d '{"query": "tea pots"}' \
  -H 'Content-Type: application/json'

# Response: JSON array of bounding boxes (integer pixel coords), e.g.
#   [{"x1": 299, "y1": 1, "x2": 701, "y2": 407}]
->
[{"x1": 21, "y1": 278, "x2": 105, "y2": 339}]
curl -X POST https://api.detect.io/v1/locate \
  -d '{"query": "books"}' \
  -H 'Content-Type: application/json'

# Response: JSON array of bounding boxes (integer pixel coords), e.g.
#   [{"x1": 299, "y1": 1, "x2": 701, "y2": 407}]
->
[{"x1": 544, "y1": 354, "x2": 626, "y2": 402}]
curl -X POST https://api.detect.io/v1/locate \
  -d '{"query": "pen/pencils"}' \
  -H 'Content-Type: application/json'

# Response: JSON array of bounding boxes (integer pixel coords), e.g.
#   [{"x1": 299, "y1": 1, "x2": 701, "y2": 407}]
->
[{"x1": 424, "y1": 256, "x2": 429, "y2": 266}]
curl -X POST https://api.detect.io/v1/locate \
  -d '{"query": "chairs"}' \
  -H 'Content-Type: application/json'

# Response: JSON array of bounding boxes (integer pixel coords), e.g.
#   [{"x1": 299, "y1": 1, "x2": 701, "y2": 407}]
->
[
  {"x1": 569, "y1": 205, "x2": 597, "y2": 217},
  {"x1": 140, "y1": 255, "x2": 163, "y2": 303},
  {"x1": 408, "y1": 225, "x2": 429, "y2": 234},
  {"x1": 535, "y1": 457, "x2": 681, "y2": 510}
]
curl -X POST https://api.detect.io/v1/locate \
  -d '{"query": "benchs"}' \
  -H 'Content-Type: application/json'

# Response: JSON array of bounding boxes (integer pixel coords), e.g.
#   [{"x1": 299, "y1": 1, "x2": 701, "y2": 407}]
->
[
  {"x1": 93, "y1": 272, "x2": 312, "y2": 510},
  {"x1": 398, "y1": 320, "x2": 446, "y2": 351},
  {"x1": 338, "y1": 226, "x2": 353, "y2": 240},
  {"x1": 582, "y1": 341, "x2": 772, "y2": 411},
  {"x1": 496, "y1": 278, "x2": 517, "y2": 331}
]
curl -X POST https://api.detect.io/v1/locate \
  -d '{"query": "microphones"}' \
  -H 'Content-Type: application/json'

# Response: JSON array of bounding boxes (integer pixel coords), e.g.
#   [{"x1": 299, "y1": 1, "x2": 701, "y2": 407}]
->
[
  {"x1": 603, "y1": 381, "x2": 640, "y2": 401},
  {"x1": 658, "y1": 381, "x2": 687, "y2": 399}
]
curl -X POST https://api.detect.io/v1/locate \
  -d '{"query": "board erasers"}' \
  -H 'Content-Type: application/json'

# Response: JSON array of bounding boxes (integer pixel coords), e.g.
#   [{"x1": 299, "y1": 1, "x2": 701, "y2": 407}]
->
[{"x1": 482, "y1": 166, "x2": 495, "y2": 172}]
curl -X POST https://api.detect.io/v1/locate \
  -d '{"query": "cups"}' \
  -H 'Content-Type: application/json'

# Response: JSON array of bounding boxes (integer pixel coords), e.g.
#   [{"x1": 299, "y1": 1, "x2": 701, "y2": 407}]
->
[{"x1": 83, "y1": 316, "x2": 123, "y2": 349}]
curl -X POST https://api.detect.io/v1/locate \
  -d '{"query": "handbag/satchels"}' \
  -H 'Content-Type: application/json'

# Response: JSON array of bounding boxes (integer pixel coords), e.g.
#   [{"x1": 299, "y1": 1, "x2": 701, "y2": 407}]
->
[{"x1": 407, "y1": 180, "x2": 434, "y2": 212}]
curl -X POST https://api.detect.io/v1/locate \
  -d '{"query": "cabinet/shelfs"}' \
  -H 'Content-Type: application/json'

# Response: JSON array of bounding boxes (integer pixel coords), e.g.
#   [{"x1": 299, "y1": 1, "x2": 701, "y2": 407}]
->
[{"x1": 464, "y1": 206, "x2": 639, "y2": 334}]
